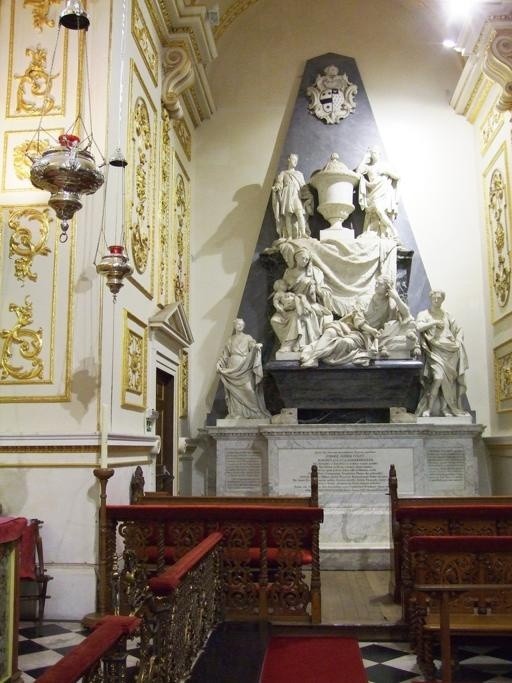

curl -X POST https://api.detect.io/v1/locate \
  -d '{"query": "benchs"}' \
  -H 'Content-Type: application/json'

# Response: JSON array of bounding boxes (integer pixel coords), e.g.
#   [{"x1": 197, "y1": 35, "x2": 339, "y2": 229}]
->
[
  {"x1": 130, "y1": 466, "x2": 323, "y2": 627},
  {"x1": 388, "y1": 464, "x2": 512, "y2": 613}
]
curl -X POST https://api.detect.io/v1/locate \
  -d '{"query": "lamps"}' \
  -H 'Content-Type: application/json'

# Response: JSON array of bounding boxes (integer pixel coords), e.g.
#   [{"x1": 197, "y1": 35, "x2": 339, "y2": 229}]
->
[{"x1": 31, "y1": 0, "x2": 133, "y2": 307}]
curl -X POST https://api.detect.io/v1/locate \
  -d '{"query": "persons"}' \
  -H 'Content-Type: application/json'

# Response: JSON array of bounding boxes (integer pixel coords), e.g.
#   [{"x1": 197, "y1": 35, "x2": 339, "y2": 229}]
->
[
  {"x1": 272, "y1": 153, "x2": 314, "y2": 242},
  {"x1": 300, "y1": 274, "x2": 409, "y2": 368},
  {"x1": 414, "y1": 289, "x2": 472, "y2": 417},
  {"x1": 356, "y1": 145, "x2": 400, "y2": 239},
  {"x1": 217, "y1": 319, "x2": 273, "y2": 419},
  {"x1": 270, "y1": 247, "x2": 331, "y2": 353},
  {"x1": 273, "y1": 280, "x2": 310, "y2": 320}
]
